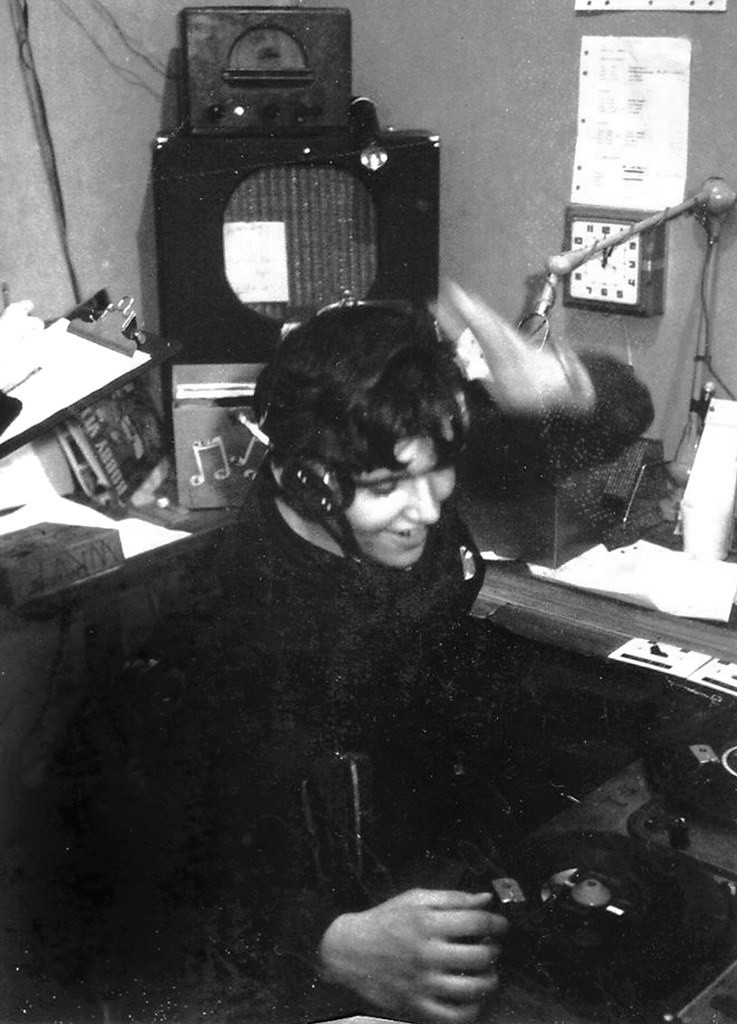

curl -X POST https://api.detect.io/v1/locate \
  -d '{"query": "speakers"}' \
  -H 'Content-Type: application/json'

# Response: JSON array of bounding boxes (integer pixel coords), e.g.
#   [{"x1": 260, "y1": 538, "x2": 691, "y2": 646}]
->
[{"x1": 152, "y1": 125, "x2": 440, "y2": 363}]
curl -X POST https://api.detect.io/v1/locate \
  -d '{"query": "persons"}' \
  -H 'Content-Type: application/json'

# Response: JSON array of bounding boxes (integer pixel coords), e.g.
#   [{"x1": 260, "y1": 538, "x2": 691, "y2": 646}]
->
[{"x1": 218, "y1": 277, "x2": 654, "y2": 1020}]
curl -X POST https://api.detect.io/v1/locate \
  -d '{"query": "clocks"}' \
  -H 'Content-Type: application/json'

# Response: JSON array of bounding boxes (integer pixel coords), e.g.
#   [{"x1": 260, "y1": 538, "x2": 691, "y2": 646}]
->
[{"x1": 562, "y1": 204, "x2": 668, "y2": 322}]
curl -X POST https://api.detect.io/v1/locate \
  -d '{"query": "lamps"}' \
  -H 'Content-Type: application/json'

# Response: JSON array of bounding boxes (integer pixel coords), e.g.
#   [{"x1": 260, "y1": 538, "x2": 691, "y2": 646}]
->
[{"x1": 518, "y1": 177, "x2": 735, "y2": 480}]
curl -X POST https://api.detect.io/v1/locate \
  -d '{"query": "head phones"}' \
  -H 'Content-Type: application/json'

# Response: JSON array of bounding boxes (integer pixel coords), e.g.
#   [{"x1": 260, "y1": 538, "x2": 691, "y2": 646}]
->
[{"x1": 277, "y1": 330, "x2": 430, "y2": 525}]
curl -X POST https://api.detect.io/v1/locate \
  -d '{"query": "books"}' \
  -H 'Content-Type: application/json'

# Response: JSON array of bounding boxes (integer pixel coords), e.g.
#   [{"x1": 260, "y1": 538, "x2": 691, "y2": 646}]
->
[
  {"x1": 175, "y1": 377, "x2": 269, "y2": 511},
  {"x1": 0, "y1": 521, "x2": 128, "y2": 606}
]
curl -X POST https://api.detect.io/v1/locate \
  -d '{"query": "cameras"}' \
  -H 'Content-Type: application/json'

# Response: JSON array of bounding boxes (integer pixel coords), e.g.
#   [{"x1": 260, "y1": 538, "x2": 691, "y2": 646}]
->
[{"x1": 540, "y1": 868, "x2": 645, "y2": 932}]
